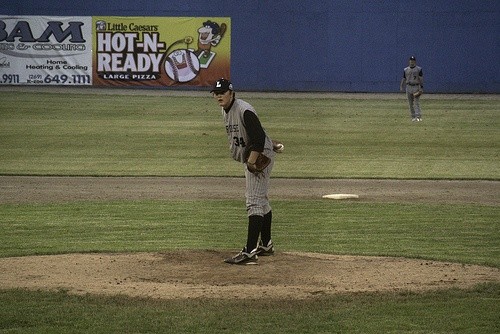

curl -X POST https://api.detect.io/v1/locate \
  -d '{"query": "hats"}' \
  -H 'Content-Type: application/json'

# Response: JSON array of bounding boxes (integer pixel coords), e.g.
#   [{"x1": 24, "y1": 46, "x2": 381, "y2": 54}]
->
[
  {"x1": 410, "y1": 56, "x2": 415, "y2": 60},
  {"x1": 210, "y1": 79, "x2": 233, "y2": 93}
]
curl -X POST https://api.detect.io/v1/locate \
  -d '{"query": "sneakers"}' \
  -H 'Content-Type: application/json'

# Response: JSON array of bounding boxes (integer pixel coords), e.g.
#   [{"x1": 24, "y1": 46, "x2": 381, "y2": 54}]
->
[
  {"x1": 224, "y1": 247, "x2": 258, "y2": 265},
  {"x1": 251, "y1": 239, "x2": 275, "y2": 255}
]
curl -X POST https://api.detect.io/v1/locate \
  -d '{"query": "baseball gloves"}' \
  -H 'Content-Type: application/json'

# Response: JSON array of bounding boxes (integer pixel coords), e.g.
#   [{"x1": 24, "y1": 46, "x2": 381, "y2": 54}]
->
[
  {"x1": 412, "y1": 89, "x2": 423, "y2": 98},
  {"x1": 247, "y1": 153, "x2": 271, "y2": 176}
]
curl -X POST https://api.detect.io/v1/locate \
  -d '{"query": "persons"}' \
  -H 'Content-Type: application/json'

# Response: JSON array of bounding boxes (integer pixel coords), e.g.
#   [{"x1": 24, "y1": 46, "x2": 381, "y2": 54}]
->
[
  {"x1": 400, "y1": 56, "x2": 424, "y2": 121},
  {"x1": 210, "y1": 78, "x2": 283, "y2": 265}
]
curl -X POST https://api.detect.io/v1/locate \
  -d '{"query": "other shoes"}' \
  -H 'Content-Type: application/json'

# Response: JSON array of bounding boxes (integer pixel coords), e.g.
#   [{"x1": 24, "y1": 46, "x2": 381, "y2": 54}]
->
[
  {"x1": 416, "y1": 117, "x2": 422, "y2": 122},
  {"x1": 411, "y1": 117, "x2": 416, "y2": 122}
]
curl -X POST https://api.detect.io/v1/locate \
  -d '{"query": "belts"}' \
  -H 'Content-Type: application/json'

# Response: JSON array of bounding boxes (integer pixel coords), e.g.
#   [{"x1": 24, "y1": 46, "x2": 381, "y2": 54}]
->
[{"x1": 407, "y1": 83, "x2": 419, "y2": 86}]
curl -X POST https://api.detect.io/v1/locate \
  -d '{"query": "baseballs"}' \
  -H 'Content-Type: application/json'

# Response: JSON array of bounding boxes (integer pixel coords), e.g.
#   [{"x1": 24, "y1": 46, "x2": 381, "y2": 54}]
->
[{"x1": 277, "y1": 144, "x2": 285, "y2": 151}]
